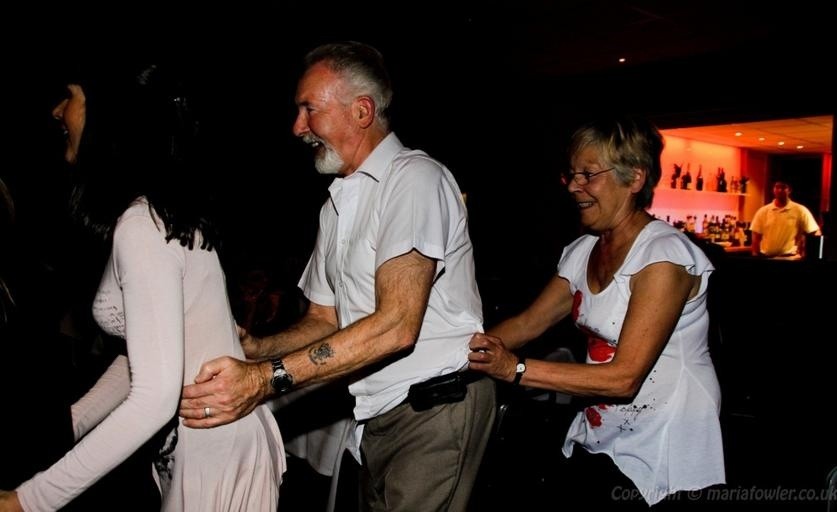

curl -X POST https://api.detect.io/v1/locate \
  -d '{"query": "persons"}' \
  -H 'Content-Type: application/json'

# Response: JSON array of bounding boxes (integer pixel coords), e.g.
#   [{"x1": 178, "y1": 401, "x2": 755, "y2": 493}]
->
[
  {"x1": 749, "y1": 181, "x2": 822, "y2": 259},
  {"x1": 0, "y1": 42, "x2": 726, "y2": 512}
]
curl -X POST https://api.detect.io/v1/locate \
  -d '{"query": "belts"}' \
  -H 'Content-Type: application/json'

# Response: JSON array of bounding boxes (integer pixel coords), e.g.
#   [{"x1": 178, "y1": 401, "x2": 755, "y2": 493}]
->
[{"x1": 462, "y1": 366, "x2": 488, "y2": 383}]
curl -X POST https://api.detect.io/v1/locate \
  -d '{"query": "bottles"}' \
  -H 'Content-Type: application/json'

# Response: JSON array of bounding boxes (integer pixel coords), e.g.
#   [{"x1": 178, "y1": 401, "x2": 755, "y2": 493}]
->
[
  {"x1": 716, "y1": 167, "x2": 744, "y2": 193},
  {"x1": 665, "y1": 213, "x2": 751, "y2": 242},
  {"x1": 683, "y1": 164, "x2": 704, "y2": 192}
]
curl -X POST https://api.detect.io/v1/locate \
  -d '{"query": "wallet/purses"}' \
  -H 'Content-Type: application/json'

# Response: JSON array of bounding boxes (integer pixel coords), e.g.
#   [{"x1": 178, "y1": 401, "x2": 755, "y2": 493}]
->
[{"x1": 408, "y1": 373, "x2": 469, "y2": 415}]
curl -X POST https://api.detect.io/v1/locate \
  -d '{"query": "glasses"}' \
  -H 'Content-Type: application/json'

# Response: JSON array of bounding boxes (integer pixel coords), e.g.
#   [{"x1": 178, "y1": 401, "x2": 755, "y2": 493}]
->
[{"x1": 561, "y1": 166, "x2": 613, "y2": 188}]
